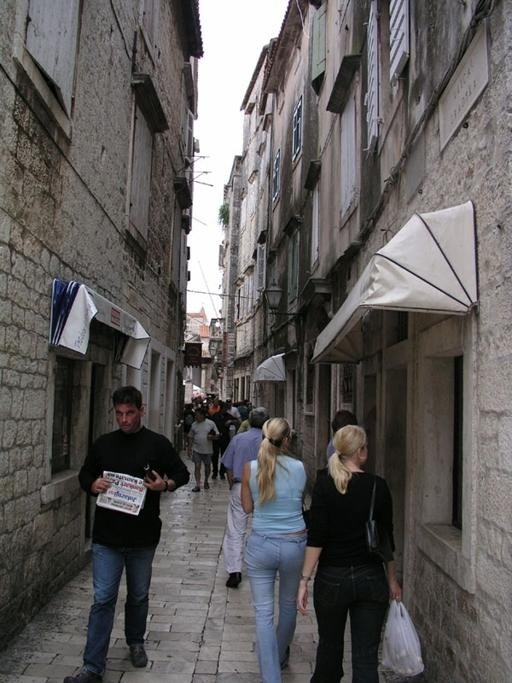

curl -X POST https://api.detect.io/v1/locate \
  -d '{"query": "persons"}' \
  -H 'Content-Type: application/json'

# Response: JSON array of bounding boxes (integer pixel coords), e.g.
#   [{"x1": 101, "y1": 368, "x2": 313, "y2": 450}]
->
[
  {"x1": 179, "y1": 392, "x2": 274, "y2": 585},
  {"x1": 238, "y1": 410, "x2": 404, "y2": 681},
  {"x1": 63, "y1": 384, "x2": 191, "y2": 682}
]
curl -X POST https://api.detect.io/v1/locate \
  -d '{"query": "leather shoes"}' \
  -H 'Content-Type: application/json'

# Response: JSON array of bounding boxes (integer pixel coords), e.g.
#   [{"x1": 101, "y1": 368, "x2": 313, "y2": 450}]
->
[
  {"x1": 191, "y1": 486, "x2": 200, "y2": 492},
  {"x1": 203, "y1": 481, "x2": 209, "y2": 489},
  {"x1": 224, "y1": 571, "x2": 241, "y2": 590}
]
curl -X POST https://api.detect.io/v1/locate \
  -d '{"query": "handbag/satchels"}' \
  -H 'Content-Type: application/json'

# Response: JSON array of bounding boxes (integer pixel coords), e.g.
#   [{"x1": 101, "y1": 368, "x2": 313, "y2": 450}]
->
[{"x1": 363, "y1": 482, "x2": 395, "y2": 563}]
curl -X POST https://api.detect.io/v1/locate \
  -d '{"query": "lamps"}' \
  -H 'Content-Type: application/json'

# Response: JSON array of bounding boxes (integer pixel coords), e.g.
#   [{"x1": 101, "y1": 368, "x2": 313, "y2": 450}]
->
[{"x1": 261, "y1": 278, "x2": 304, "y2": 316}]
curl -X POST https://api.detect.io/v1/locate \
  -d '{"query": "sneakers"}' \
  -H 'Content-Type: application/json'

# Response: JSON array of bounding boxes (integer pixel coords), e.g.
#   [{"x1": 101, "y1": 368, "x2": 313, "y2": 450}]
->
[
  {"x1": 63, "y1": 666, "x2": 103, "y2": 683},
  {"x1": 127, "y1": 641, "x2": 149, "y2": 668}
]
